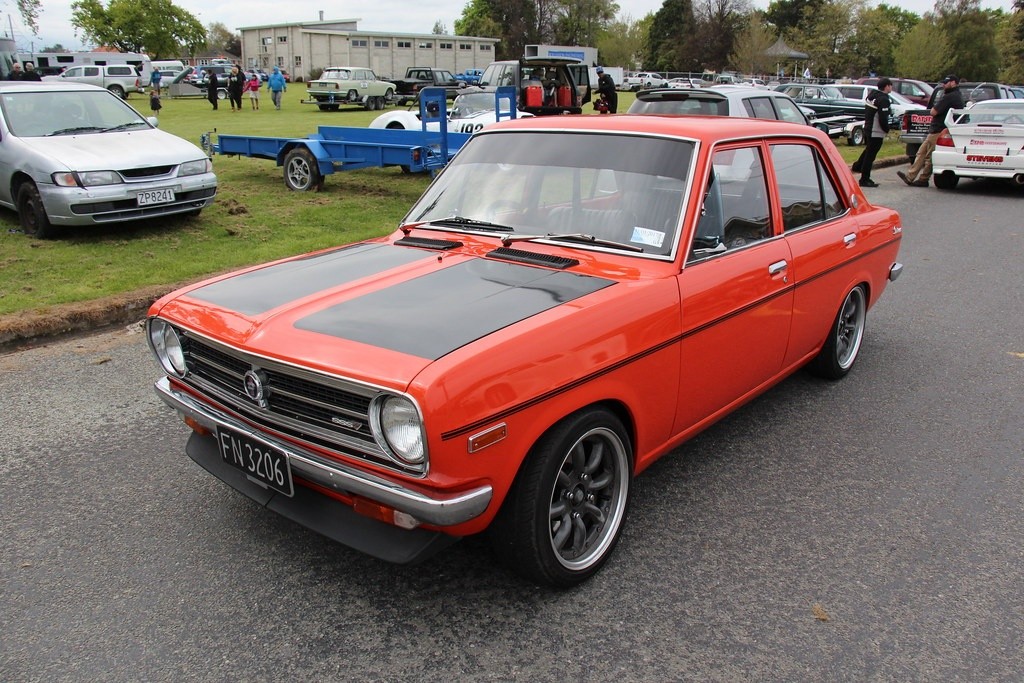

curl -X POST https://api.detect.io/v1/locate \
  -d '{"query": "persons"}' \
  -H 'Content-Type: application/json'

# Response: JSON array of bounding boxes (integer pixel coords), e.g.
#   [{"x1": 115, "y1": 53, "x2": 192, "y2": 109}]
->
[
  {"x1": 149, "y1": 66, "x2": 162, "y2": 99},
  {"x1": 227, "y1": 64, "x2": 287, "y2": 112},
  {"x1": 206, "y1": 69, "x2": 220, "y2": 110},
  {"x1": 896, "y1": 75, "x2": 965, "y2": 187},
  {"x1": 149, "y1": 90, "x2": 162, "y2": 116},
  {"x1": 851, "y1": 78, "x2": 894, "y2": 188},
  {"x1": 5, "y1": 63, "x2": 42, "y2": 81},
  {"x1": 592, "y1": 66, "x2": 618, "y2": 114}
]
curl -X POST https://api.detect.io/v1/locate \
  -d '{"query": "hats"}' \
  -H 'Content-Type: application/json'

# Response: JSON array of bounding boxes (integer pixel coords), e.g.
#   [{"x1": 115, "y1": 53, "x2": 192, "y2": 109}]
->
[
  {"x1": 231, "y1": 67, "x2": 239, "y2": 75},
  {"x1": 596, "y1": 66, "x2": 603, "y2": 74},
  {"x1": 940, "y1": 75, "x2": 959, "y2": 84},
  {"x1": 26, "y1": 62, "x2": 34, "y2": 70}
]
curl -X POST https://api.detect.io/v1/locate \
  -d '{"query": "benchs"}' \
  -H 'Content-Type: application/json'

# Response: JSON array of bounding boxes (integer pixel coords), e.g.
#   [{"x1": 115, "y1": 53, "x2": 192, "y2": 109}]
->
[{"x1": 623, "y1": 173, "x2": 771, "y2": 223}]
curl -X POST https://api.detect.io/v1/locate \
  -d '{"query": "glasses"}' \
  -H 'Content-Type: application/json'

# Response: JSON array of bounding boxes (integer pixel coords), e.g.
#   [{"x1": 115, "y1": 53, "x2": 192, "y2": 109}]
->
[{"x1": 597, "y1": 71, "x2": 603, "y2": 74}]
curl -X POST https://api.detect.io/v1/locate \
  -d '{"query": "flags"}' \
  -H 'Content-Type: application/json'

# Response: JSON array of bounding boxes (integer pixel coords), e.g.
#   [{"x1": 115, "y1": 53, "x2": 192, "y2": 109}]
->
[{"x1": 803, "y1": 68, "x2": 811, "y2": 80}]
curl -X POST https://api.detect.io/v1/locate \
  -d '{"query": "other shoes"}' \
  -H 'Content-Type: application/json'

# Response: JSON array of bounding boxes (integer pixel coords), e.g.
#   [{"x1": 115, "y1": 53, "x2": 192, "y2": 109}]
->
[
  {"x1": 910, "y1": 179, "x2": 929, "y2": 187},
  {"x1": 858, "y1": 177, "x2": 879, "y2": 187},
  {"x1": 896, "y1": 170, "x2": 913, "y2": 186}
]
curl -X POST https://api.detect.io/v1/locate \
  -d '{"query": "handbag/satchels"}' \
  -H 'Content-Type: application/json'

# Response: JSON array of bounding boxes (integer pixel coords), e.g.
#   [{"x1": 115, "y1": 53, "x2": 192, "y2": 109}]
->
[{"x1": 593, "y1": 98, "x2": 610, "y2": 111}]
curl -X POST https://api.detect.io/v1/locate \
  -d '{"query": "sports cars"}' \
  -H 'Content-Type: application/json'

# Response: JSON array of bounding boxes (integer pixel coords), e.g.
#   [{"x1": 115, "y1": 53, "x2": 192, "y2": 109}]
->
[{"x1": 369, "y1": 89, "x2": 537, "y2": 176}]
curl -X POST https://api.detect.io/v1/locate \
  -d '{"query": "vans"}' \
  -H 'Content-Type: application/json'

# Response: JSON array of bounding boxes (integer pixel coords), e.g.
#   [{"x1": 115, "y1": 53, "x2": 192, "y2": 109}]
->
[{"x1": 476, "y1": 56, "x2": 593, "y2": 116}]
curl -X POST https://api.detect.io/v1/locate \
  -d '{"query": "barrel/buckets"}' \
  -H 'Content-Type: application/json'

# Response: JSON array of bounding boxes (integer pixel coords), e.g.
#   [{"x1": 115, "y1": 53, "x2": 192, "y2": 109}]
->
[
  {"x1": 558, "y1": 86, "x2": 571, "y2": 107},
  {"x1": 527, "y1": 86, "x2": 542, "y2": 106}
]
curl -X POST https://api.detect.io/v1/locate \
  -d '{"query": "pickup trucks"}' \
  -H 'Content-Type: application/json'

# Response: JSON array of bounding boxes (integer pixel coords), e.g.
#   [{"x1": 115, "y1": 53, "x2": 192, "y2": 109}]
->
[
  {"x1": 381, "y1": 65, "x2": 469, "y2": 106},
  {"x1": 898, "y1": 82, "x2": 1016, "y2": 167},
  {"x1": 40, "y1": 62, "x2": 146, "y2": 101},
  {"x1": 448, "y1": 68, "x2": 484, "y2": 86}
]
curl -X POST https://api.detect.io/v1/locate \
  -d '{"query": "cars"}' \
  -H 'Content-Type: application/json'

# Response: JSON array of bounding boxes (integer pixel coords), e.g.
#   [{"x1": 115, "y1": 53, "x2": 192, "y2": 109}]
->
[
  {"x1": 279, "y1": 69, "x2": 291, "y2": 84},
  {"x1": 143, "y1": 107, "x2": 904, "y2": 587},
  {"x1": 0, "y1": 79, "x2": 218, "y2": 240},
  {"x1": 622, "y1": 70, "x2": 1024, "y2": 130},
  {"x1": 149, "y1": 59, "x2": 269, "y2": 100},
  {"x1": 931, "y1": 96, "x2": 1024, "y2": 196},
  {"x1": 611, "y1": 84, "x2": 831, "y2": 194},
  {"x1": 306, "y1": 67, "x2": 397, "y2": 103}
]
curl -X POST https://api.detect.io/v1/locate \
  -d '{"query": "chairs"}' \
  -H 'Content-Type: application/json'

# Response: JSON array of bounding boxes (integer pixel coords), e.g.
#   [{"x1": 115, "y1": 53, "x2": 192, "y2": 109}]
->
[
  {"x1": 451, "y1": 105, "x2": 473, "y2": 118},
  {"x1": 508, "y1": 71, "x2": 525, "y2": 85},
  {"x1": 543, "y1": 71, "x2": 556, "y2": 87},
  {"x1": 530, "y1": 69, "x2": 544, "y2": 81},
  {"x1": 687, "y1": 107, "x2": 705, "y2": 115},
  {"x1": 55, "y1": 102, "x2": 82, "y2": 129},
  {"x1": 420, "y1": 75, "x2": 426, "y2": 79},
  {"x1": 549, "y1": 206, "x2": 632, "y2": 243}
]
